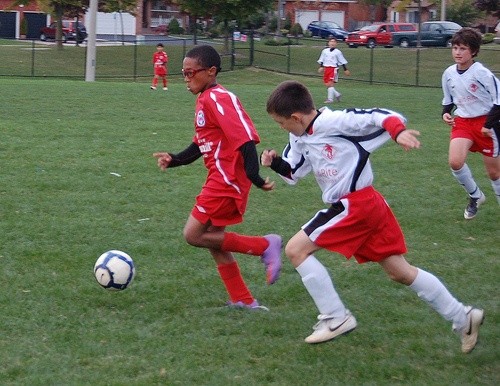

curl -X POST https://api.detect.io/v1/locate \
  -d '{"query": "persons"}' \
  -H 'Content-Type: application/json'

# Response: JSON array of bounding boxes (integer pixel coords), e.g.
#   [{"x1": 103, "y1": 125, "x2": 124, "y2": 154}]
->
[
  {"x1": 152, "y1": 45, "x2": 284, "y2": 311},
  {"x1": 441, "y1": 28, "x2": 500, "y2": 220},
  {"x1": 317, "y1": 38, "x2": 350, "y2": 104},
  {"x1": 150, "y1": 43, "x2": 169, "y2": 91},
  {"x1": 260, "y1": 80, "x2": 485, "y2": 354}
]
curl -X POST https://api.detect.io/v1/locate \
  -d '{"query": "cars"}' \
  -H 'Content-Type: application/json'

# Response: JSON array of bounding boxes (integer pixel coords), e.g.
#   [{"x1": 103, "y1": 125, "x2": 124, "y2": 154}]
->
[{"x1": 308, "y1": 20, "x2": 348, "y2": 41}]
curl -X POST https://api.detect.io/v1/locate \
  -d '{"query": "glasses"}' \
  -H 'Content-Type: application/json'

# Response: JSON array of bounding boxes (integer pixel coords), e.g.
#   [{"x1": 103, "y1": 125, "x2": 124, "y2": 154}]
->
[{"x1": 180, "y1": 65, "x2": 210, "y2": 79}]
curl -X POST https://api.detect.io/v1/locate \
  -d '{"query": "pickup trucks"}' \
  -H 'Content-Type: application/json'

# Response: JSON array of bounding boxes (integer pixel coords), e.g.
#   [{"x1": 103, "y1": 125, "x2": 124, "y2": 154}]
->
[
  {"x1": 391, "y1": 21, "x2": 463, "y2": 49},
  {"x1": 345, "y1": 23, "x2": 417, "y2": 48}
]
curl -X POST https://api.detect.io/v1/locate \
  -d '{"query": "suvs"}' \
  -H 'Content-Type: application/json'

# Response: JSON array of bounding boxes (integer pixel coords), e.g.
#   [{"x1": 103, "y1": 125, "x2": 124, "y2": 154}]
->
[{"x1": 39, "y1": 20, "x2": 88, "y2": 44}]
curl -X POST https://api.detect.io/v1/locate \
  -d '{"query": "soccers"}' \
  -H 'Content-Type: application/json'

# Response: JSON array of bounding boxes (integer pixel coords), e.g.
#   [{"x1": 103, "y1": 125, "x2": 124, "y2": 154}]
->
[{"x1": 94, "y1": 250, "x2": 135, "y2": 291}]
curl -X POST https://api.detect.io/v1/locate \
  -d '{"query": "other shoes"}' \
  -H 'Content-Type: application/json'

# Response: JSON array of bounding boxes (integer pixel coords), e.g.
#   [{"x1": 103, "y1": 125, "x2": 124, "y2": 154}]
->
[
  {"x1": 162, "y1": 87, "x2": 169, "y2": 91},
  {"x1": 226, "y1": 298, "x2": 260, "y2": 309},
  {"x1": 336, "y1": 94, "x2": 341, "y2": 102},
  {"x1": 323, "y1": 98, "x2": 334, "y2": 103},
  {"x1": 463, "y1": 192, "x2": 486, "y2": 220},
  {"x1": 260, "y1": 233, "x2": 284, "y2": 286},
  {"x1": 150, "y1": 86, "x2": 157, "y2": 90}
]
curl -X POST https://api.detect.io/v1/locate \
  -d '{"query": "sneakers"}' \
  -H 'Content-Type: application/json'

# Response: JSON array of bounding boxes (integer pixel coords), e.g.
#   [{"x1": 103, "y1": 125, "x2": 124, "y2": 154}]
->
[
  {"x1": 461, "y1": 309, "x2": 485, "y2": 353},
  {"x1": 304, "y1": 311, "x2": 358, "y2": 345}
]
